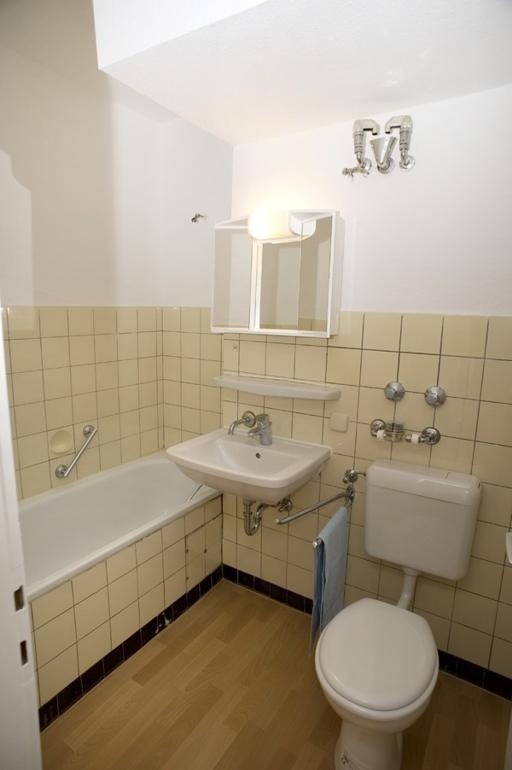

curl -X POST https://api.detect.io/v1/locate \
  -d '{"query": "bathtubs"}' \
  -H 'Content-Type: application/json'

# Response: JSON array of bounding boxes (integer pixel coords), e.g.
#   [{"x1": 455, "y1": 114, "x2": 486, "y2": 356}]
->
[{"x1": 17, "y1": 448, "x2": 224, "y2": 601}]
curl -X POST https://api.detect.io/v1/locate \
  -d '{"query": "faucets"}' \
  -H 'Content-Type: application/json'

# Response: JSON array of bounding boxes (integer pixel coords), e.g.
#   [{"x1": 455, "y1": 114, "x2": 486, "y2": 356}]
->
[
  {"x1": 246, "y1": 414, "x2": 272, "y2": 443},
  {"x1": 229, "y1": 412, "x2": 251, "y2": 438}
]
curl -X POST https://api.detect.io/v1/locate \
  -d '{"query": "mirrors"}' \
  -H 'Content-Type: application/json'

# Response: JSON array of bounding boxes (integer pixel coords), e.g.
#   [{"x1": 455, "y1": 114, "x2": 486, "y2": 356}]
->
[
  {"x1": 212, "y1": 214, "x2": 332, "y2": 333},
  {"x1": 309, "y1": 506, "x2": 349, "y2": 647}
]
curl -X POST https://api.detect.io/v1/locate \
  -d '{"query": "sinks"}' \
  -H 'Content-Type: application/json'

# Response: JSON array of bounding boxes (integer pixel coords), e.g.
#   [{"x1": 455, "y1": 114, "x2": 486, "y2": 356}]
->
[{"x1": 167, "y1": 427, "x2": 330, "y2": 504}]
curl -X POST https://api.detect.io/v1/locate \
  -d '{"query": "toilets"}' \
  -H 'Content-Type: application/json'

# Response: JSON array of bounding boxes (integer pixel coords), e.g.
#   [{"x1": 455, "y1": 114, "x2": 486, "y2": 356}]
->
[{"x1": 314, "y1": 457, "x2": 481, "y2": 768}]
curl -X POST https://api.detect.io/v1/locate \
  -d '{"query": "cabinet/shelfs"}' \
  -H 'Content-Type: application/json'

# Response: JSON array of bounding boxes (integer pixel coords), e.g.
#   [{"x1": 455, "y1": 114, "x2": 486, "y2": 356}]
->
[{"x1": 211, "y1": 208, "x2": 344, "y2": 340}]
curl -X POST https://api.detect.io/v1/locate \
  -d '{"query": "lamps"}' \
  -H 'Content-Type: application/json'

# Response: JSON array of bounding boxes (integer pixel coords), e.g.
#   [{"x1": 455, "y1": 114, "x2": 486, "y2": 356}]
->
[{"x1": 247, "y1": 211, "x2": 302, "y2": 239}]
